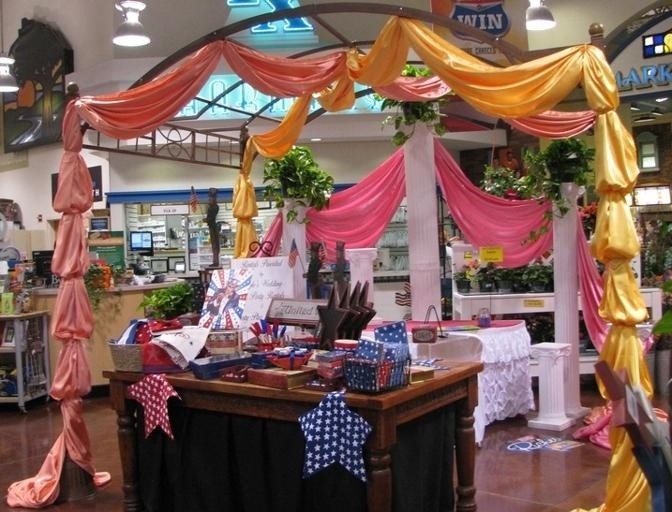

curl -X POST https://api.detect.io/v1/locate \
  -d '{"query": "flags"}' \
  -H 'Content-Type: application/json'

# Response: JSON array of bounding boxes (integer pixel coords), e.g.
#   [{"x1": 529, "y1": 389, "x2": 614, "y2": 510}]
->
[{"x1": 191, "y1": 187, "x2": 197, "y2": 212}]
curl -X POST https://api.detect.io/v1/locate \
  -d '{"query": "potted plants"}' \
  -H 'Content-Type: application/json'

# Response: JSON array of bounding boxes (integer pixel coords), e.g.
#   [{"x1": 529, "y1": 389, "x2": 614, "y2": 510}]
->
[
  {"x1": 370, "y1": 64, "x2": 457, "y2": 147},
  {"x1": 515, "y1": 138, "x2": 596, "y2": 245},
  {"x1": 452, "y1": 260, "x2": 554, "y2": 293},
  {"x1": 86, "y1": 264, "x2": 126, "y2": 312},
  {"x1": 261, "y1": 143, "x2": 337, "y2": 224}
]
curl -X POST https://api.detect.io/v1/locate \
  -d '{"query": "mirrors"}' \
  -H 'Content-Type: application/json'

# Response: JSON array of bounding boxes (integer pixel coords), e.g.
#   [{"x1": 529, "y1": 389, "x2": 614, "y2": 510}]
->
[{"x1": 112, "y1": 0, "x2": 151, "y2": 47}]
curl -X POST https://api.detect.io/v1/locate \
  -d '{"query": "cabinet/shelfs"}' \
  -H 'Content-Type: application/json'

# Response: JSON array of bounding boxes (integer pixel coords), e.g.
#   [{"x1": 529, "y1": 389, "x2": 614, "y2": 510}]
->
[{"x1": 0, "y1": 310, "x2": 52, "y2": 414}]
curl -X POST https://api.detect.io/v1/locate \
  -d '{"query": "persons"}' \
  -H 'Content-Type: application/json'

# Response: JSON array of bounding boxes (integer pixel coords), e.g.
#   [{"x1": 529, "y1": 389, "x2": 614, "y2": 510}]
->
[
  {"x1": 440, "y1": 230, "x2": 453, "y2": 298},
  {"x1": 203, "y1": 187, "x2": 220, "y2": 266}
]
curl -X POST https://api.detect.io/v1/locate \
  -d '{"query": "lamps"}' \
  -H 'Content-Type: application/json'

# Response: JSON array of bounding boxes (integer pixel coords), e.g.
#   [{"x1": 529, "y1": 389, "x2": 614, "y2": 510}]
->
[{"x1": 525, "y1": 0, "x2": 555, "y2": 31}]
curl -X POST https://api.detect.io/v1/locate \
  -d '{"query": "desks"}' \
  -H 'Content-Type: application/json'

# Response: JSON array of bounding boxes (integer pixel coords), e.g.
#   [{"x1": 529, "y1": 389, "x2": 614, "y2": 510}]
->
[
  {"x1": 362, "y1": 319, "x2": 536, "y2": 427},
  {"x1": 103, "y1": 359, "x2": 485, "y2": 512},
  {"x1": 452, "y1": 244, "x2": 662, "y2": 375}
]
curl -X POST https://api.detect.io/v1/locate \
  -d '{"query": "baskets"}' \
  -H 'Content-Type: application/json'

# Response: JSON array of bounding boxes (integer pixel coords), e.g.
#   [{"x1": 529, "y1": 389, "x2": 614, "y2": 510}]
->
[
  {"x1": 343, "y1": 350, "x2": 411, "y2": 394},
  {"x1": 107, "y1": 342, "x2": 192, "y2": 373}
]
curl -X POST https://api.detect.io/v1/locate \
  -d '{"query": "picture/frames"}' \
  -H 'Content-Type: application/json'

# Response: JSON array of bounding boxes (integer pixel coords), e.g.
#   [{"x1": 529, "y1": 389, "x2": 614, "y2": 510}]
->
[{"x1": 151, "y1": 256, "x2": 185, "y2": 273}]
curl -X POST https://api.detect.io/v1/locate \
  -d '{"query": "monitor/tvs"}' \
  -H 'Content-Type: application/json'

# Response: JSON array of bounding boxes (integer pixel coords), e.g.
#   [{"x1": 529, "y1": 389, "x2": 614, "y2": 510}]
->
[
  {"x1": 130, "y1": 231, "x2": 153, "y2": 250},
  {"x1": 32, "y1": 251, "x2": 54, "y2": 288}
]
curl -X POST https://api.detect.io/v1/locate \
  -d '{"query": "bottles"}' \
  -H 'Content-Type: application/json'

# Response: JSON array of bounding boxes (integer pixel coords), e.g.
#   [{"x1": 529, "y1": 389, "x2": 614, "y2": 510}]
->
[{"x1": 479, "y1": 305, "x2": 491, "y2": 328}]
